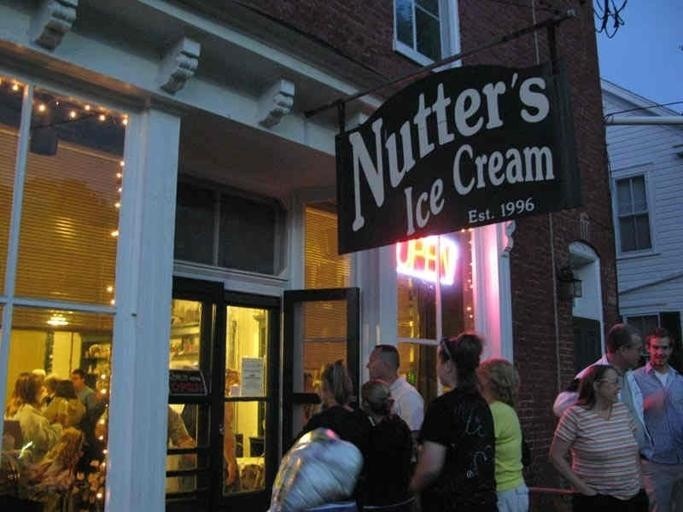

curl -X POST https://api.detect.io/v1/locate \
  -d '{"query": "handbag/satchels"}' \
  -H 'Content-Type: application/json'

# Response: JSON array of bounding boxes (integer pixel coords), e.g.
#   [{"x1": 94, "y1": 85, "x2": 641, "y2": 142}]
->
[{"x1": 521, "y1": 439, "x2": 532, "y2": 465}]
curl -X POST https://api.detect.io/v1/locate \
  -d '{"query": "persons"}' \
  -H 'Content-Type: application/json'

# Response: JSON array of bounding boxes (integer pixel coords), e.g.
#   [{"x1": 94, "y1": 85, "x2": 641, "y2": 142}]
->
[
  {"x1": 4, "y1": 369, "x2": 96, "y2": 452},
  {"x1": 366, "y1": 344, "x2": 424, "y2": 454},
  {"x1": 632, "y1": 328, "x2": 682, "y2": 512},
  {"x1": 352, "y1": 380, "x2": 417, "y2": 511},
  {"x1": 411, "y1": 333, "x2": 497, "y2": 511},
  {"x1": 222, "y1": 394, "x2": 240, "y2": 494},
  {"x1": 296, "y1": 360, "x2": 374, "y2": 445},
  {"x1": 552, "y1": 323, "x2": 654, "y2": 465},
  {"x1": 547, "y1": 364, "x2": 650, "y2": 511},
  {"x1": 165, "y1": 405, "x2": 197, "y2": 463},
  {"x1": 475, "y1": 359, "x2": 530, "y2": 511}
]
